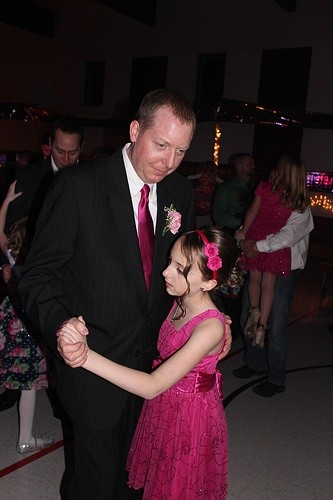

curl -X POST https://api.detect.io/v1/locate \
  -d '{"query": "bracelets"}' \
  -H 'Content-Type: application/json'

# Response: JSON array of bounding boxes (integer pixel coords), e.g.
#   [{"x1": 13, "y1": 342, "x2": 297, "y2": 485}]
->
[{"x1": 237, "y1": 238, "x2": 244, "y2": 249}]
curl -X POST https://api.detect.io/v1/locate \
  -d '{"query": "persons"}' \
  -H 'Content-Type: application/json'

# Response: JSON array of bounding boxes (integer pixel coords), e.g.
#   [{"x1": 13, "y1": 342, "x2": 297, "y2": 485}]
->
[
  {"x1": 10, "y1": 91, "x2": 233, "y2": 500},
  {"x1": 58, "y1": 228, "x2": 247, "y2": 500},
  {"x1": 0, "y1": 117, "x2": 315, "y2": 456}
]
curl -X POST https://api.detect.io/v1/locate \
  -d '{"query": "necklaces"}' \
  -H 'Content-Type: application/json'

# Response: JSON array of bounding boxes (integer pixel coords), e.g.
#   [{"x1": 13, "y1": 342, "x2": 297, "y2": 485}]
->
[{"x1": 170, "y1": 298, "x2": 210, "y2": 323}]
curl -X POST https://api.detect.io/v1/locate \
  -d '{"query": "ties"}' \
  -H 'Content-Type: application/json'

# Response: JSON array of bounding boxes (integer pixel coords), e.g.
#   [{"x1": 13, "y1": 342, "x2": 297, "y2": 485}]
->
[{"x1": 138, "y1": 184, "x2": 155, "y2": 290}]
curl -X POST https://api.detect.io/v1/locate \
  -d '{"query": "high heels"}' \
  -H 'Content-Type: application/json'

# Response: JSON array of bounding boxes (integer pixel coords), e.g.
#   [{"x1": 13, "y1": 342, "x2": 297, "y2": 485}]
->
[
  {"x1": 251, "y1": 326, "x2": 264, "y2": 348},
  {"x1": 242, "y1": 307, "x2": 259, "y2": 339}
]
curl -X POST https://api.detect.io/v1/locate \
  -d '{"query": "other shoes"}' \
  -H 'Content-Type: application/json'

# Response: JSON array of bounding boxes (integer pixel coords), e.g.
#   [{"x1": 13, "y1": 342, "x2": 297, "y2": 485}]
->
[
  {"x1": 252, "y1": 381, "x2": 284, "y2": 397},
  {"x1": 232, "y1": 363, "x2": 257, "y2": 378},
  {"x1": 17, "y1": 436, "x2": 55, "y2": 453}
]
